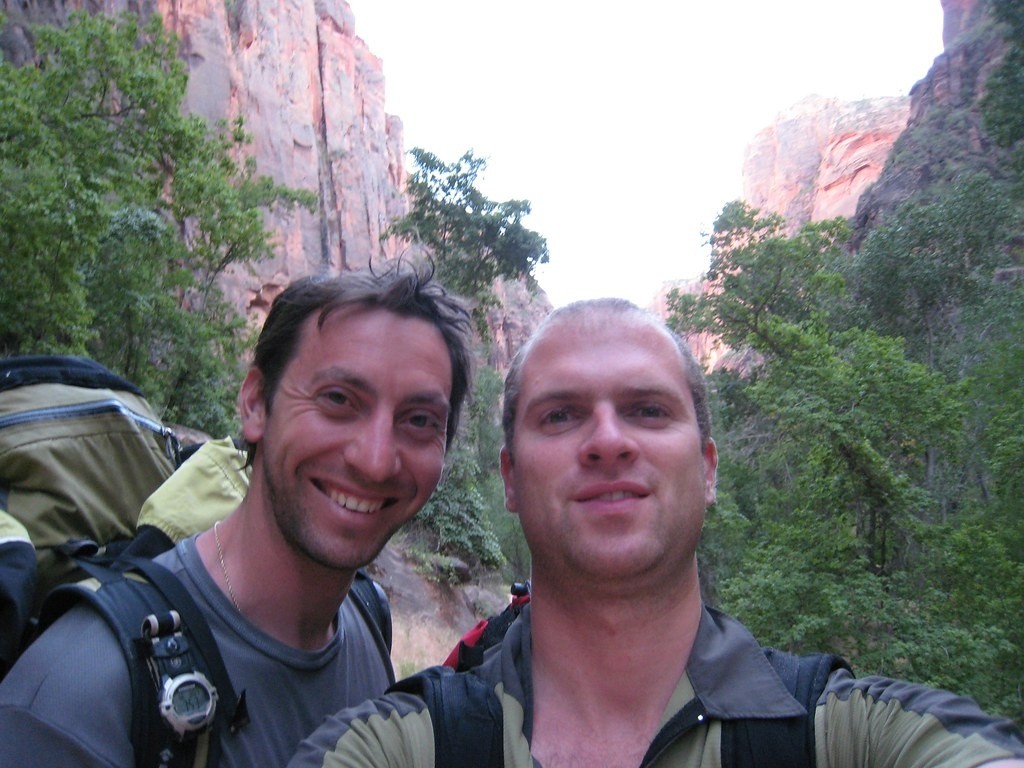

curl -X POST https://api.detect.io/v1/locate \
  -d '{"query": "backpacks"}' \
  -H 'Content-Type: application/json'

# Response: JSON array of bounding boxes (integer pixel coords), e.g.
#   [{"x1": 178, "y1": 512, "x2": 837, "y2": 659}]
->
[
  {"x1": 0, "y1": 355, "x2": 396, "y2": 768},
  {"x1": 384, "y1": 580, "x2": 856, "y2": 768}
]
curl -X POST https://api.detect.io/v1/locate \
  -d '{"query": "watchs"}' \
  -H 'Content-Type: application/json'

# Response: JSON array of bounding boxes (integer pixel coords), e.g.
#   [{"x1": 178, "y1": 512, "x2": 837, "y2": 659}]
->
[{"x1": 140, "y1": 633, "x2": 219, "y2": 768}]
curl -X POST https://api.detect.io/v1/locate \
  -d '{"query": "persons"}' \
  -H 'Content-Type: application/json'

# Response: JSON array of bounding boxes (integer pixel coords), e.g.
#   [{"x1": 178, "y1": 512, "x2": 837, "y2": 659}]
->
[
  {"x1": 287, "y1": 296, "x2": 1024, "y2": 768},
  {"x1": 0, "y1": 245, "x2": 473, "y2": 768}
]
